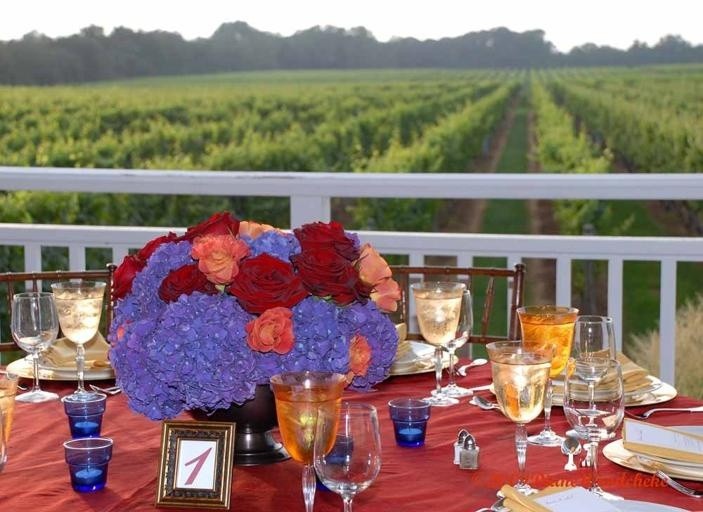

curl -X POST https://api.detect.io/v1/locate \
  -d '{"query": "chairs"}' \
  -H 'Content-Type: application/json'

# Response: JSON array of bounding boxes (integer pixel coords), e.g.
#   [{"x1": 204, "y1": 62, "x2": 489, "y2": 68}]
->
[
  {"x1": 389, "y1": 263, "x2": 525, "y2": 357},
  {"x1": 0, "y1": 264, "x2": 117, "y2": 352}
]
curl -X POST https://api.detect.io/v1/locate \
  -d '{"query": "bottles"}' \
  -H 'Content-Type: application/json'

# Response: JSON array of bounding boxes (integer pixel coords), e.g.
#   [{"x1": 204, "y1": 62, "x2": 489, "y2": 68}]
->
[{"x1": 454, "y1": 429, "x2": 479, "y2": 469}]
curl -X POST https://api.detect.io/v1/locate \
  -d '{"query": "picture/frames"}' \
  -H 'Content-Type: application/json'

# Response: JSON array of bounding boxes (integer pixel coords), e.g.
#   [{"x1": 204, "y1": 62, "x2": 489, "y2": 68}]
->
[{"x1": 155, "y1": 420, "x2": 236, "y2": 511}]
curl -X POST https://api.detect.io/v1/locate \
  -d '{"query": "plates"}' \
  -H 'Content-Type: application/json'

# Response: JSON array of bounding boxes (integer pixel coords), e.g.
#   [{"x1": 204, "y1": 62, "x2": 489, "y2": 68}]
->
[
  {"x1": 390, "y1": 341, "x2": 458, "y2": 376},
  {"x1": 7, "y1": 353, "x2": 120, "y2": 382},
  {"x1": 603, "y1": 426, "x2": 702, "y2": 481},
  {"x1": 625, "y1": 382, "x2": 677, "y2": 407}
]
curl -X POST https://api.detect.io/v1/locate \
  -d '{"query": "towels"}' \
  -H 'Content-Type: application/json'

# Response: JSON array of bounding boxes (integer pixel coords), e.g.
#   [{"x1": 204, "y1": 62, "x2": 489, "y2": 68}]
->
[
  {"x1": 41, "y1": 331, "x2": 111, "y2": 373},
  {"x1": 573, "y1": 349, "x2": 654, "y2": 395},
  {"x1": 390, "y1": 321, "x2": 413, "y2": 359}
]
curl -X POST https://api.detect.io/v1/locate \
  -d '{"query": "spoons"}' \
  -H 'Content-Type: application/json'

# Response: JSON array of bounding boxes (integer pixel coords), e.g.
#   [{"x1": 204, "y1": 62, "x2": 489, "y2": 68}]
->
[
  {"x1": 472, "y1": 396, "x2": 498, "y2": 414},
  {"x1": 476, "y1": 496, "x2": 512, "y2": 512}
]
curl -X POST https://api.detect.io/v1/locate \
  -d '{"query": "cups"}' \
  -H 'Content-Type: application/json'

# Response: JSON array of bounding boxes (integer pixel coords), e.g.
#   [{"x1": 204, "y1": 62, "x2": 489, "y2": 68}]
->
[
  {"x1": 0, "y1": 373, "x2": 18, "y2": 465},
  {"x1": 388, "y1": 398, "x2": 431, "y2": 448},
  {"x1": 64, "y1": 396, "x2": 114, "y2": 492}
]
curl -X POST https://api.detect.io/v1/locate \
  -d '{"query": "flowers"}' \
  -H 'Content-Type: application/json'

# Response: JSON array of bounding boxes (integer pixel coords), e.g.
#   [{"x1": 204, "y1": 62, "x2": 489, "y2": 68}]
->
[{"x1": 105, "y1": 212, "x2": 401, "y2": 422}]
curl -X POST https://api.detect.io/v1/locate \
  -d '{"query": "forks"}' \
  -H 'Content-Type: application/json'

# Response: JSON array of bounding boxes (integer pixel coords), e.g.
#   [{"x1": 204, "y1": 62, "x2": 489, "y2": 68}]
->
[
  {"x1": 624, "y1": 406, "x2": 703, "y2": 420},
  {"x1": 656, "y1": 469, "x2": 703, "y2": 500},
  {"x1": 92, "y1": 381, "x2": 122, "y2": 396},
  {"x1": 447, "y1": 358, "x2": 487, "y2": 377}
]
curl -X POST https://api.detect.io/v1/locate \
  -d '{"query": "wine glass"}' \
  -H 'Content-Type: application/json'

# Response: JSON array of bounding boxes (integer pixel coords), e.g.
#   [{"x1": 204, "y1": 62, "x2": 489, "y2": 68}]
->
[
  {"x1": 11, "y1": 281, "x2": 107, "y2": 403},
  {"x1": 485, "y1": 304, "x2": 625, "y2": 495},
  {"x1": 267, "y1": 372, "x2": 381, "y2": 512},
  {"x1": 410, "y1": 281, "x2": 473, "y2": 406}
]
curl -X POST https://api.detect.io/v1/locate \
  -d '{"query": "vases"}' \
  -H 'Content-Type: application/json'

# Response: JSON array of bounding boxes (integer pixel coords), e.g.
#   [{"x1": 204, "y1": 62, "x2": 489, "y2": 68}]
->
[{"x1": 184, "y1": 384, "x2": 292, "y2": 466}]
